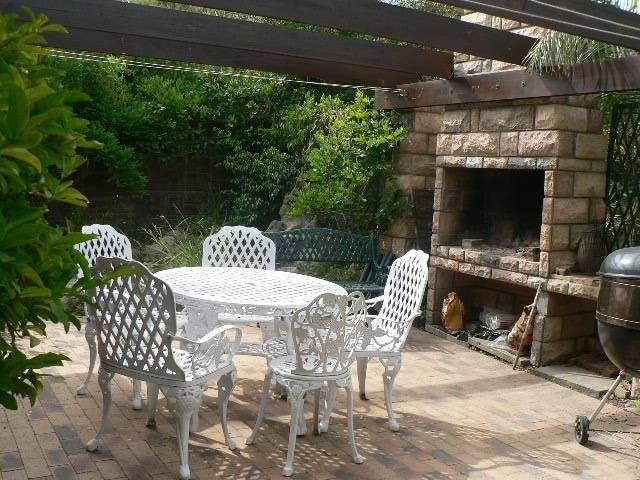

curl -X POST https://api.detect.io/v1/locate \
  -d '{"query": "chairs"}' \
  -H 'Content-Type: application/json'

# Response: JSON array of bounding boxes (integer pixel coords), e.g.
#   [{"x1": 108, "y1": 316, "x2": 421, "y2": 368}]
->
[
  {"x1": 87, "y1": 255, "x2": 242, "y2": 477},
  {"x1": 319, "y1": 249, "x2": 430, "y2": 432},
  {"x1": 244, "y1": 291, "x2": 367, "y2": 476},
  {"x1": 201, "y1": 226, "x2": 277, "y2": 271},
  {"x1": 73, "y1": 227, "x2": 187, "y2": 411}
]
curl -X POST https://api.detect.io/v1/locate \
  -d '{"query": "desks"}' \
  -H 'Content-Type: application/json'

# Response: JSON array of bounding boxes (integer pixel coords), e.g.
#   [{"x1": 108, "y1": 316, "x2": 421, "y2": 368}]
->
[{"x1": 150, "y1": 266, "x2": 348, "y2": 435}]
